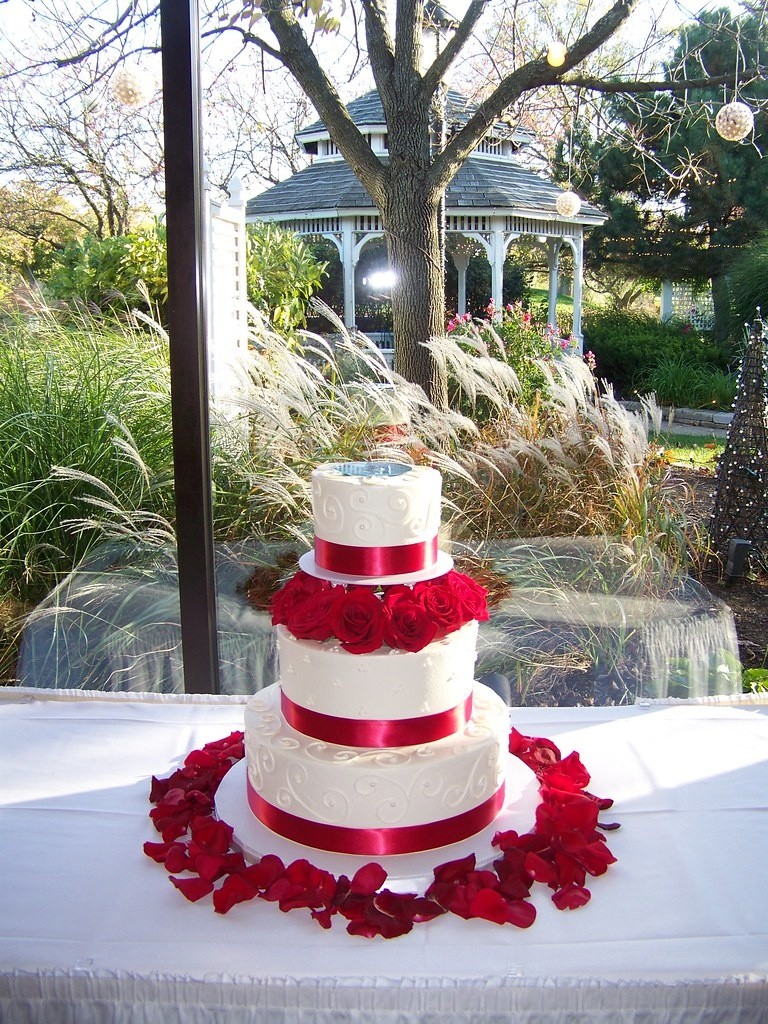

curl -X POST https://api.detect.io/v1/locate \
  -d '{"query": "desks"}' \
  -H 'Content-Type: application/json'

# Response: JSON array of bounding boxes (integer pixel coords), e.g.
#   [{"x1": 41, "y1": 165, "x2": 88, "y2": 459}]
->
[{"x1": 0, "y1": 683, "x2": 768, "y2": 1024}]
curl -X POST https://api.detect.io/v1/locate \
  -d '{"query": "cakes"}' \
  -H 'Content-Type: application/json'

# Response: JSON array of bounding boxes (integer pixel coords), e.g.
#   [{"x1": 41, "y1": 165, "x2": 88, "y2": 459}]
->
[{"x1": 244, "y1": 462, "x2": 512, "y2": 856}]
